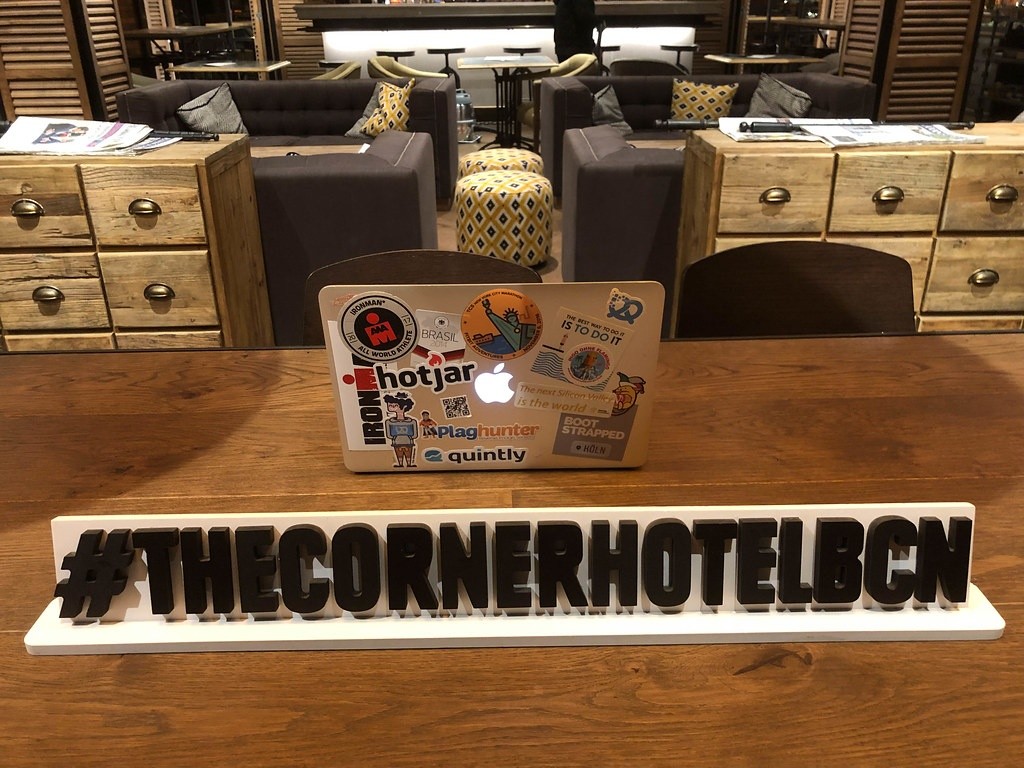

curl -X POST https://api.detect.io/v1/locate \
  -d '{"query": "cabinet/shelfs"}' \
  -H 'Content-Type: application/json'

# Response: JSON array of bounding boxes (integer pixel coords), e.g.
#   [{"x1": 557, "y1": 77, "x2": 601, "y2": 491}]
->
[
  {"x1": 1, "y1": 128, "x2": 277, "y2": 352},
  {"x1": 672, "y1": 119, "x2": 1024, "y2": 332}
]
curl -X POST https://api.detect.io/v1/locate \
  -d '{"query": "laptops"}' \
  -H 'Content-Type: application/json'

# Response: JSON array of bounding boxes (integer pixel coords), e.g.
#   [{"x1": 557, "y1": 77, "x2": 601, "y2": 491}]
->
[{"x1": 318, "y1": 282, "x2": 664, "y2": 473}]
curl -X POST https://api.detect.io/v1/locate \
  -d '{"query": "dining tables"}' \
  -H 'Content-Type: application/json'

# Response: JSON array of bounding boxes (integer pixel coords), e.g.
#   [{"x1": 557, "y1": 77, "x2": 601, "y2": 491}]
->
[
  {"x1": 165, "y1": 56, "x2": 291, "y2": 82},
  {"x1": 706, "y1": 51, "x2": 825, "y2": 73}
]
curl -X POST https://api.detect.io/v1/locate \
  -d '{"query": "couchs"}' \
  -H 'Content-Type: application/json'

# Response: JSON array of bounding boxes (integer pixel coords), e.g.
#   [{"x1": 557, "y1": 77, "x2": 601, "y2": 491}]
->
[{"x1": 111, "y1": 73, "x2": 890, "y2": 347}]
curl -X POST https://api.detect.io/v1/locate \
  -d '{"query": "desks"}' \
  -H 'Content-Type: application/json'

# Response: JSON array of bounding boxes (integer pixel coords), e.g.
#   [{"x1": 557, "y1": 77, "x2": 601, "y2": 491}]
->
[{"x1": 294, "y1": 0, "x2": 753, "y2": 70}]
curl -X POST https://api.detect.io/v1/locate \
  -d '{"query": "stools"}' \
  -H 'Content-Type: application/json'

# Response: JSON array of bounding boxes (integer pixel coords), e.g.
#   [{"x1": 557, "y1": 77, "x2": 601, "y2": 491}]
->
[
  {"x1": 375, "y1": 51, "x2": 415, "y2": 63},
  {"x1": 426, "y1": 47, "x2": 468, "y2": 91},
  {"x1": 504, "y1": 46, "x2": 542, "y2": 118},
  {"x1": 593, "y1": 43, "x2": 622, "y2": 76},
  {"x1": 320, "y1": 56, "x2": 354, "y2": 68},
  {"x1": 452, "y1": 169, "x2": 554, "y2": 268},
  {"x1": 458, "y1": 146, "x2": 547, "y2": 175},
  {"x1": 661, "y1": 43, "x2": 701, "y2": 75}
]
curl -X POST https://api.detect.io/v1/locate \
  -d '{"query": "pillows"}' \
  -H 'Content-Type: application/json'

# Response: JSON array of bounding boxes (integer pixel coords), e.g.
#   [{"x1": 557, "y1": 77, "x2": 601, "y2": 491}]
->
[
  {"x1": 345, "y1": 77, "x2": 417, "y2": 140},
  {"x1": 666, "y1": 76, "x2": 739, "y2": 125},
  {"x1": 176, "y1": 82, "x2": 251, "y2": 138},
  {"x1": 591, "y1": 83, "x2": 634, "y2": 139},
  {"x1": 741, "y1": 70, "x2": 814, "y2": 118}
]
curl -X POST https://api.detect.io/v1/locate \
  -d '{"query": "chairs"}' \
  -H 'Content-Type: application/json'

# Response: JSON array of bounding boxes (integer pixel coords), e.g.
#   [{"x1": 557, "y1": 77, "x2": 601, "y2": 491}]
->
[{"x1": 610, "y1": 57, "x2": 687, "y2": 75}]
